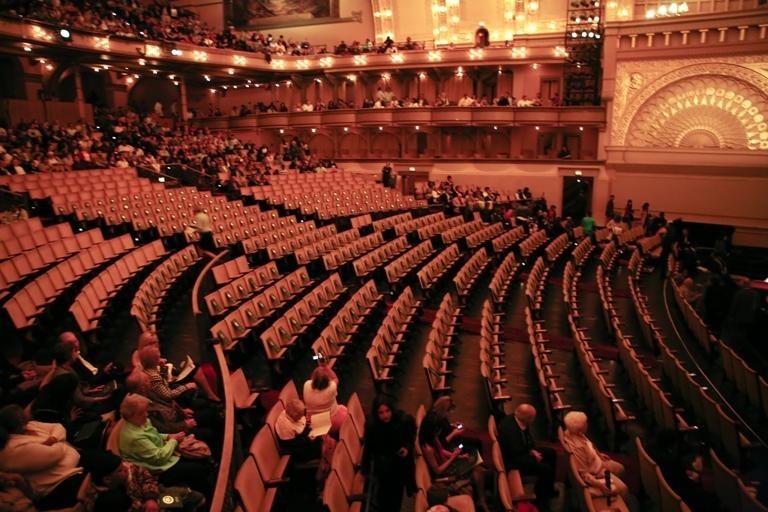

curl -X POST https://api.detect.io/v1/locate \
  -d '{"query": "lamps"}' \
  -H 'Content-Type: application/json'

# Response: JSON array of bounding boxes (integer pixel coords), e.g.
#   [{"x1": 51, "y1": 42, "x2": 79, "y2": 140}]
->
[{"x1": 559, "y1": 1, "x2": 605, "y2": 107}]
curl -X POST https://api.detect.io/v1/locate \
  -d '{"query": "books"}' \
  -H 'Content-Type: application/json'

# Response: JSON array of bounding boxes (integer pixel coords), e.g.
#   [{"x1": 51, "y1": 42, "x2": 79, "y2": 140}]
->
[{"x1": 308, "y1": 410, "x2": 333, "y2": 439}]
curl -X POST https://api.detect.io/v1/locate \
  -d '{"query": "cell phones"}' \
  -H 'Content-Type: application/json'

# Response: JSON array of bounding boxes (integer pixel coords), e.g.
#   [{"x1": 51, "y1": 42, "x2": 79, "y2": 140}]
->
[
  {"x1": 457, "y1": 424, "x2": 462, "y2": 429},
  {"x1": 458, "y1": 443, "x2": 463, "y2": 448}
]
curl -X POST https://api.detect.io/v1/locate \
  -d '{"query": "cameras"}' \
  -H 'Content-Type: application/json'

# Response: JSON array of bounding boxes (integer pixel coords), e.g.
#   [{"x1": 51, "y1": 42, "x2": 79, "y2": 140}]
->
[{"x1": 312, "y1": 353, "x2": 325, "y2": 363}]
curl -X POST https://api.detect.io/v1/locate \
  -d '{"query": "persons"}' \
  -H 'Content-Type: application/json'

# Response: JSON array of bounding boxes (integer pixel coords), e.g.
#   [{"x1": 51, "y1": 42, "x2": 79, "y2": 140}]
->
[
  {"x1": 274, "y1": 398, "x2": 336, "y2": 461},
  {"x1": 303, "y1": 359, "x2": 348, "y2": 436},
  {"x1": 330, "y1": 25, "x2": 602, "y2": 107},
  {"x1": 359, "y1": 398, "x2": 418, "y2": 498},
  {"x1": 564, "y1": 411, "x2": 635, "y2": 508},
  {"x1": 1, "y1": 329, "x2": 225, "y2": 511},
  {"x1": 644, "y1": 427, "x2": 717, "y2": 512},
  {"x1": 418, "y1": 395, "x2": 491, "y2": 512},
  {"x1": 0, "y1": 1, "x2": 338, "y2": 200},
  {"x1": 498, "y1": 403, "x2": 561, "y2": 510},
  {"x1": 381, "y1": 160, "x2": 734, "y2": 293},
  {"x1": 188, "y1": 205, "x2": 219, "y2": 254}
]
